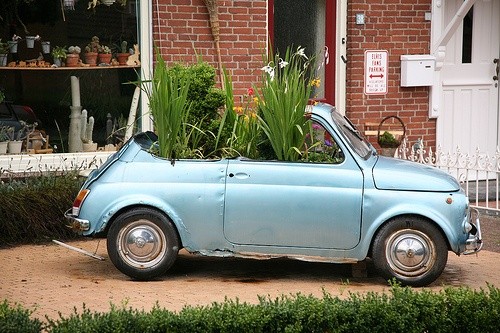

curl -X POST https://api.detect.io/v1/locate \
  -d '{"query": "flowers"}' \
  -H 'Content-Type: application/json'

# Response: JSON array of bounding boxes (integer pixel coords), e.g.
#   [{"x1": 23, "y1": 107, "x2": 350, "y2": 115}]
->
[
  {"x1": 67, "y1": 46, "x2": 81, "y2": 53},
  {"x1": 26, "y1": 33, "x2": 42, "y2": 41},
  {"x1": 235, "y1": 42, "x2": 336, "y2": 153},
  {"x1": 12, "y1": 34, "x2": 22, "y2": 41}
]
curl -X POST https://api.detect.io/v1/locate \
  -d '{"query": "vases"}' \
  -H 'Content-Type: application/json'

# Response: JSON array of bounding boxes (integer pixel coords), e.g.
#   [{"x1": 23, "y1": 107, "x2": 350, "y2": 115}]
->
[
  {"x1": 26, "y1": 36, "x2": 35, "y2": 48},
  {"x1": 41, "y1": 40, "x2": 50, "y2": 53},
  {"x1": 67, "y1": 54, "x2": 79, "y2": 67},
  {"x1": 8, "y1": 40, "x2": 18, "y2": 54}
]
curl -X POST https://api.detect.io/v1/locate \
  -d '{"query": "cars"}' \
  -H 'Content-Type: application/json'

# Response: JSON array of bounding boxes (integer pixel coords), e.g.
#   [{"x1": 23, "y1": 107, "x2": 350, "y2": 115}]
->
[{"x1": 62, "y1": 101, "x2": 484, "y2": 288}]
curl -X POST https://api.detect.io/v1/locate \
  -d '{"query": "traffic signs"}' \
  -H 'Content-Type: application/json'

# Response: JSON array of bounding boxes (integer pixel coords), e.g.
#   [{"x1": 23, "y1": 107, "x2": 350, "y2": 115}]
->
[{"x1": 365, "y1": 48, "x2": 388, "y2": 95}]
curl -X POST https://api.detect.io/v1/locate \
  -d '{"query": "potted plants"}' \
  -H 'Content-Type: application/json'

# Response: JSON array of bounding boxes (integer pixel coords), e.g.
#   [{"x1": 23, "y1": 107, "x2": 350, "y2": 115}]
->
[
  {"x1": 379, "y1": 131, "x2": 398, "y2": 157},
  {"x1": 0, "y1": 39, "x2": 9, "y2": 66},
  {"x1": 51, "y1": 45, "x2": 66, "y2": 67},
  {"x1": 0, "y1": 120, "x2": 38, "y2": 154},
  {"x1": 98, "y1": 45, "x2": 112, "y2": 64},
  {"x1": 84, "y1": 36, "x2": 99, "y2": 66},
  {"x1": 116, "y1": 41, "x2": 131, "y2": 65},
  {"x1": 80, "y1": 109, "x2": 97, "y2": 152}
]
